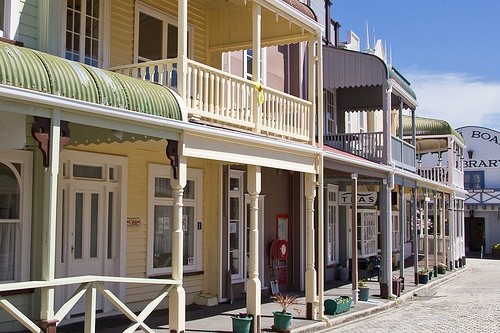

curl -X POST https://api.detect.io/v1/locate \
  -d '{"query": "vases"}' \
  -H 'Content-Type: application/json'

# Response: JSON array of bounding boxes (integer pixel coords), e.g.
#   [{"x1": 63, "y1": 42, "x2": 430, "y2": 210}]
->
[
  {"x1": 429, "y1": 272, "x2": 433, "y2": 280},
  {"x1": 438, "y1": 268, "x2": 446, "y2": 274},
  {"x1": 392, "y1": 281, "x2": 401, "y2": 297},
  {"x1": 419, "y1": 274, "x2": 428, "y2": 284},
  {"x1": 230, "y1": 316, "x2": 253, "y2": 333},
  {"x1": 324, "y1": 296, "x2": 352, "y2": 315},
  {"x1": 492, "y1": 248, "x2": 500, "y2": 258}
]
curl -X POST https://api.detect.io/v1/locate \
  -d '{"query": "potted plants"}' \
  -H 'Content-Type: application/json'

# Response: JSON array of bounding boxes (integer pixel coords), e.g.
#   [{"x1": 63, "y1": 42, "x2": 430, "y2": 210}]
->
[
  {"x1": 271, "y1": 292, "x2": 301, "y2": 333},
  {"x1": 358, "y1": 280, "x2": 370, "y2": 301}
]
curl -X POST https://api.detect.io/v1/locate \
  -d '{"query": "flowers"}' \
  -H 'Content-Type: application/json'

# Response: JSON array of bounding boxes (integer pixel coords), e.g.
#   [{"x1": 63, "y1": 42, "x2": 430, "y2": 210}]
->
[
  {"x1": 393, "y1": 275, "x2": 402, "y2": 281},
  {"x1": 334, "y1": 297, "x2": 350, "y2": 303},
  {"x1": 493, "y1": 243, "x2": 500, "y2": 248},
  {"x1": 438, "y1": 263, "x2": 448, "y2": 269},
  {"x1": 239, "y1": 313, "x2": 248, "y2": 318},
  {"x1": 428, "y1": 269, "x2": 433, "y2": 272},
  {"x1": 419, "y1": 267, "x2": 428, "y2": 274}
]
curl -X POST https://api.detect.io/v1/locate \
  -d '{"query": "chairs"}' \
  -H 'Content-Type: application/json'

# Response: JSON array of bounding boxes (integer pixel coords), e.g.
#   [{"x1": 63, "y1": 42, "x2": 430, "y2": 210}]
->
[{"x1": 368, "y1": 255, "x2": 381, "y2": 281}]
